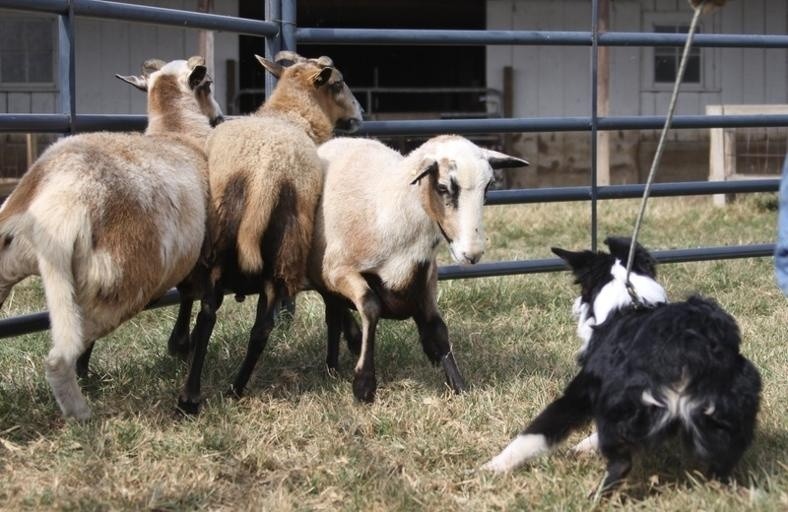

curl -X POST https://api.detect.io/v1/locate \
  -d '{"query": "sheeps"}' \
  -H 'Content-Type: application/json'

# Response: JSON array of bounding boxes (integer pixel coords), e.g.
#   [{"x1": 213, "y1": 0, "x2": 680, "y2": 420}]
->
[
  {"x1": 304, "y1": 133, "x2": 529, "y2": 408},
  {"x1": 166, "y1": 49, "x2": 365, "y2": 418},
  {"x1": 0, "y1": 54, "x2": 225, "y2": 421}
]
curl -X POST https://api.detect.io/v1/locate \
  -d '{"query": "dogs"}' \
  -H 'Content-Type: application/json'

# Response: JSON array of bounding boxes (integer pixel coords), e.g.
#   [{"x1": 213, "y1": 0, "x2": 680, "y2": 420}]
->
[{"x1": 478, "y1": 236, "x2": 764, "y2": 503}]
